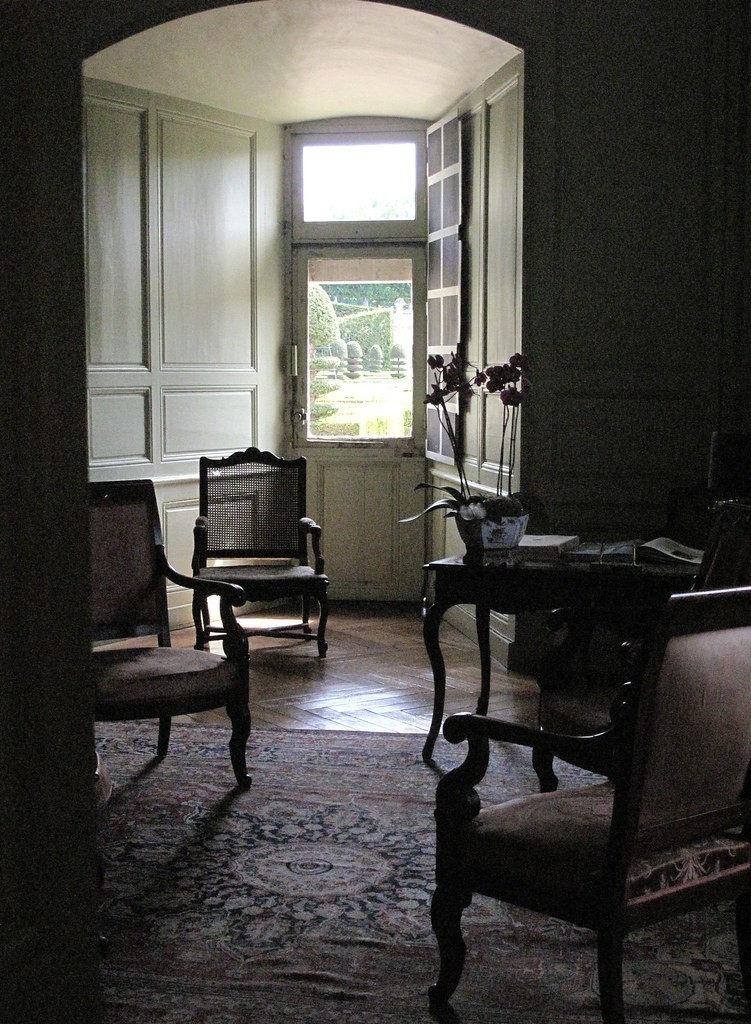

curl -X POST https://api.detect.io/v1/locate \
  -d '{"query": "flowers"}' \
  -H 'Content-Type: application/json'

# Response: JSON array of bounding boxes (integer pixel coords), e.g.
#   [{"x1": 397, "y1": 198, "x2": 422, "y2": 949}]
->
[{"x1": 398, "y1": 349, "x2": 536, "y2": 524}]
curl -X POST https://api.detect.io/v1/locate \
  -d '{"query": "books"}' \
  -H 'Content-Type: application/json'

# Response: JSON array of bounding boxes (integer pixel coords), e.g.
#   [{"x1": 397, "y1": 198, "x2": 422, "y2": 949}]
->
[{"x1": 514, "y1": 535, "x2": 706, "y2": 565}]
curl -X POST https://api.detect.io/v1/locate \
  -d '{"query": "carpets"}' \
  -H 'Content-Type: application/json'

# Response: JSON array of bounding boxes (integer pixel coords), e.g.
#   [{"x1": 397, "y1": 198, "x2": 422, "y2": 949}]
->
[{"x1": 93, "y1": 721, "x2": 751, "y2": 1024}]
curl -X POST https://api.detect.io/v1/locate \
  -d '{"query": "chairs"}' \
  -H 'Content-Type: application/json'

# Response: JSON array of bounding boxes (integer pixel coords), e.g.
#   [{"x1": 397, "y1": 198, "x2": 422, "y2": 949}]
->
[
  {"x1": 531, "y1": 500, "x2": 751, "y2": 792},
  {"x1": 190, "y1": 447, "x2": 331, "y2": 661},
  {"x1": 428, "y1": 585, "x2": 751, "y2": 1024},
  {"x1": 87, "y1": 479, "x2": 255, "y2": 791}
]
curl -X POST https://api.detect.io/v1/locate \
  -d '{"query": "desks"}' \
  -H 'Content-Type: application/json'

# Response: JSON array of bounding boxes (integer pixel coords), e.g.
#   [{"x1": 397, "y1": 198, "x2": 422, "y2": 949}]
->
[{"x1": 421, "y1": 556, "x2": 700, "y2": 766}]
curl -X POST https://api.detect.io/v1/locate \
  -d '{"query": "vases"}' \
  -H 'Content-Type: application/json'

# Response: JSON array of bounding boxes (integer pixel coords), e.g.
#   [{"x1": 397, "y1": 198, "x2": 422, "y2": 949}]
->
[{"x1": 453, "y1": 514, "x2": 530, "y2": 549}]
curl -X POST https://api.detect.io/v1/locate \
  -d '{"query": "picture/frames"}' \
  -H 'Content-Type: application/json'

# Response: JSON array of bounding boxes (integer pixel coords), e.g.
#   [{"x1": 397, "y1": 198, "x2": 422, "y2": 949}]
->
[{"x1": 427, "y1": 343, "x2": 463, "y2": 416}]
